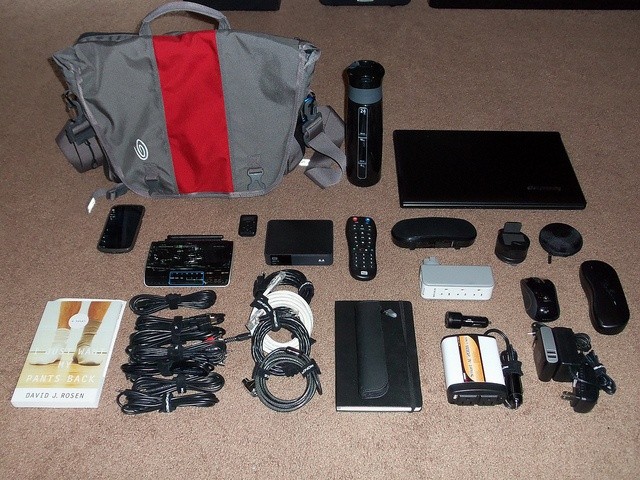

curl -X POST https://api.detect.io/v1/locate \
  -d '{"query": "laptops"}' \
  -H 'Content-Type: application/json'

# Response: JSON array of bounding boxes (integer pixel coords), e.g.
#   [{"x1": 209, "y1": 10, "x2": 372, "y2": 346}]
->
[{"x1": 393, "y1": 130, "x2": 587, "y2": 210}]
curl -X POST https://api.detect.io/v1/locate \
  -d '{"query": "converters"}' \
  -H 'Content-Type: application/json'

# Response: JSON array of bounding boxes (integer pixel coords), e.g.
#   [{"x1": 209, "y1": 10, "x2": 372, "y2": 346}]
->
[
  {"x1": 532, "y1": 326, "x2": 558, "y2": 382},
  {"x1": 560, "y1": 361, "x2": 597, "y2": 414},
  {"x1": 552, "y1": 327, "x2": 579, "y2": 382}
]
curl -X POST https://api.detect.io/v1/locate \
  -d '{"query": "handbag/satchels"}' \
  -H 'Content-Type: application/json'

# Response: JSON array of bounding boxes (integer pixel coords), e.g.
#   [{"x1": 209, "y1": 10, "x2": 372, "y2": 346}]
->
[{"x1": 52, "y1": 2, "x2": 347, "y2": 198}]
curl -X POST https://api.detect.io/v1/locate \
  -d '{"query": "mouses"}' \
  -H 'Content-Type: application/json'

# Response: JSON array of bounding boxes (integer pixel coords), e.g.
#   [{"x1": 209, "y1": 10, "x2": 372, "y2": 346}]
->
[{"x1": 520, "y1": 277, "x2": 560, "y2": 322}]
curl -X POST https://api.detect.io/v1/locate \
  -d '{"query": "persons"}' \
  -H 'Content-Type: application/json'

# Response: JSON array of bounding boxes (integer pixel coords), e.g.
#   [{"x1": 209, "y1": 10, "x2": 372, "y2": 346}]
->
[{"x1": 29, "y1": 301, "x2": 111, "y2": 365}]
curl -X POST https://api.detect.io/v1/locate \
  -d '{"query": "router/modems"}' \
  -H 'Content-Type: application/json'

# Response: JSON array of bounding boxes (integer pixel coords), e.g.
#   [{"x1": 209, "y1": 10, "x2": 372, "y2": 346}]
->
[{"x1": 265, "y1": 218, "x2": 334, "y2": 266}]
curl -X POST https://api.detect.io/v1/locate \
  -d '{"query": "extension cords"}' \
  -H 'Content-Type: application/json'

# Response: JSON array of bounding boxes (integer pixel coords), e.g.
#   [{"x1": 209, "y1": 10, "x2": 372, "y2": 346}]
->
[{"x1": 419, "y1": 256, "x2": 495, "y2": 300}]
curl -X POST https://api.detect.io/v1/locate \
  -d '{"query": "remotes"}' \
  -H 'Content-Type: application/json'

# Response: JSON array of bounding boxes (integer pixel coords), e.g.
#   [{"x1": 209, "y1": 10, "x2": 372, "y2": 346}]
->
[{"x1": 345, "y1": 216, "x2": 377, "y2": 280}]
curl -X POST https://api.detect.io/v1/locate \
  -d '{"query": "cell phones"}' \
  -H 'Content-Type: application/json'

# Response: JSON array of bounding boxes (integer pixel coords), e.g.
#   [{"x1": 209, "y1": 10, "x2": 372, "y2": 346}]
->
[
  {"x1": 239, "y1": 215, "x2": 257, "y2": 236},
  {"x1": 97, "y1": 204, "x2": 145, "y2": 254}
]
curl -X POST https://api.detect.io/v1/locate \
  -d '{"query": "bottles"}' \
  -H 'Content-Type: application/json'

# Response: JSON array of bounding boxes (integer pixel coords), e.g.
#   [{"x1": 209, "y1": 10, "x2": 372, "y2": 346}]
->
[{"x1": 345, "y1": 59, "x2": 386, "y2": 187}]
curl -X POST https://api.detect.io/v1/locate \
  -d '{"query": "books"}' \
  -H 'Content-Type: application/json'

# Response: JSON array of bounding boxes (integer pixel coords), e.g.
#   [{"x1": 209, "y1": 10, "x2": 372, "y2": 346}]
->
[
  {"x1": 335, "y1": 301, "x2": 423, "y2": 413},
  {"x1": 10, "y1": 298, "x2": 127, "y2": 408}
]
